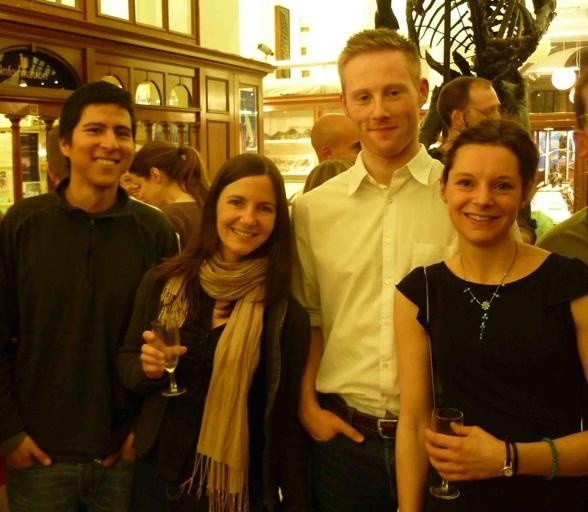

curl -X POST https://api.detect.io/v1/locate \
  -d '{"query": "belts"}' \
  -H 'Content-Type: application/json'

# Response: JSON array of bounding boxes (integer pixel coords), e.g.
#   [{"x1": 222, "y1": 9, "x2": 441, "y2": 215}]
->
[{"x1": 327, "y1": 396, "x2": 397, "y2": 441}]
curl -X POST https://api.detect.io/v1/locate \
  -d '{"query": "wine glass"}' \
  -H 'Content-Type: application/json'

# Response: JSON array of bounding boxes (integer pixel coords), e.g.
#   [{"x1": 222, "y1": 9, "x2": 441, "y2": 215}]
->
[
  {"x1": 150, "y1": 318, "x2": 191, "y2": 397},
  {"x1": 430, "y1": 407, "x2": 464, "y2": 501}
]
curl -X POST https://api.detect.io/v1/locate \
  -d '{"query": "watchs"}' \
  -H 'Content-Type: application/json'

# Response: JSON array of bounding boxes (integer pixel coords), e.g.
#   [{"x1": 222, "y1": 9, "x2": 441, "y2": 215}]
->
[{"x1": 503, "y1": 439, "x2": 513, "y2": 482}]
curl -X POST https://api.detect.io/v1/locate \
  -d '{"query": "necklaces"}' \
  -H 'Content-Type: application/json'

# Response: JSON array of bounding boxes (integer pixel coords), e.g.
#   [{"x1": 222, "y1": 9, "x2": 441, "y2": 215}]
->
[{"x1": 455, "y1": 242, "x2": 521, "y2": 345}]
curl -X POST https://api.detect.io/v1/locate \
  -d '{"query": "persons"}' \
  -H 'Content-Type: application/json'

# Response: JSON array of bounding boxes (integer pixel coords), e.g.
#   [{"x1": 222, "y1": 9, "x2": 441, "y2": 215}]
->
[
  {"x1": 310, "y1": 113, "x2": 365, "y2": 173},
  {"x1": 536, "y1": 70, "x2": 587, "y2": 264},
  {"x1": 304, "y1": 157, "x2": 355, "y2": 196},
  {"x1": 118, "y1": 150, "x2": 144, "y2": 197},
  {"x1": 286, "y1": 28, "x2": 528, "y2": 509},
  {"x1": 548, "y1": 154, "x2": 566, "y2": 179},
  {"x1": 128, "y1": 138, "x2": 211, "y2": 254},
  {"x1": 387, "y1": 119, "x2": 587, "y2": 512},
  {"x1": 43, "y1": 125, "x2": 69, "y2": 188},
  {"x1": 114, "y1": 147, "x2": 322, "y2": 512},
  {"x1": 4, "y1": 80, "x2": 182, "y2": 511},
  {"x1": 424, "y1": 75, "x2": 507, "y2": 168}
]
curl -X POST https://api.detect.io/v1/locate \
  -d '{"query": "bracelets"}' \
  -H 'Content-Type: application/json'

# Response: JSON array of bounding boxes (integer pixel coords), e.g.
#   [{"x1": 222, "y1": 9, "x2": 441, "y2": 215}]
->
[
  {"x1": 510, "y1": 441, "x2": 519, "y2": 478},
  {"x1": 541, "y1": 434, "x2": 559, "y2": 483}
]
